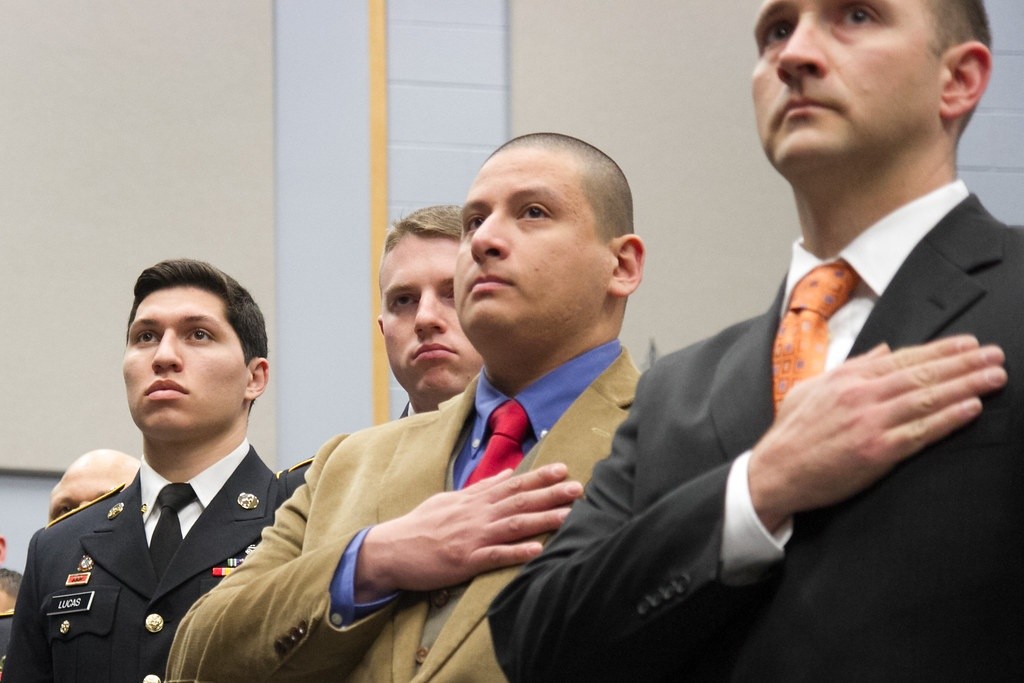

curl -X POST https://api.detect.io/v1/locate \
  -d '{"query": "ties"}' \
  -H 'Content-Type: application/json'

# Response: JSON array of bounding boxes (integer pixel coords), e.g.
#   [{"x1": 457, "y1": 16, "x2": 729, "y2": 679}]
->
[
  {"x1": 463, "y1": 399, "x2": 530, "y2": 490},
  {"x1": 772, "y1": 260, "x2": 862, "y2": 424},
  {"x1": 149, "y1": 483, "x2": 197, "y2": 582}
]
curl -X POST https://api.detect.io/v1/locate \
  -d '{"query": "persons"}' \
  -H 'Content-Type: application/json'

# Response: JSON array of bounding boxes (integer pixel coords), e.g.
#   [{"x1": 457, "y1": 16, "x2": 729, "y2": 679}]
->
[
  {"x1": 164, "y1": 132, "x2": 644, "y2": 683},
  {"x1": 377, "y1": 204, "x2": 484, "y2": 419},
  {"x1": 0, "y1": 259, "x2": 318, "y2": 683},
  {"x1": 485, "y1": 2, "x2": 1024, "y2": 683}
]
